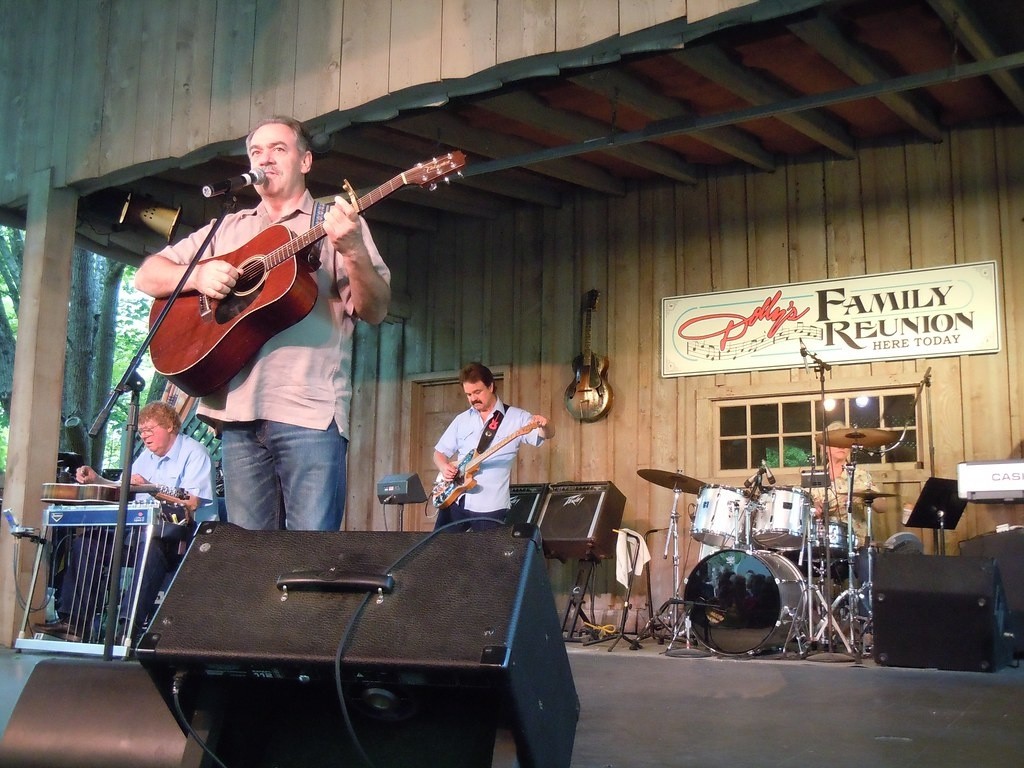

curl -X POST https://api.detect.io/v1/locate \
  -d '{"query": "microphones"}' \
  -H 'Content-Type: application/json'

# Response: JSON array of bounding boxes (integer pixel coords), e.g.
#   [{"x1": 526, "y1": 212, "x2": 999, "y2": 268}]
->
[
  {"x1": 762, "y1": 460, "x2": 776, "y2": 485},
  {"x1": 203, "y1": 168, "x2": 267, "y2": 197},
  {"x1": 744, "y1": 469, "x2": 764, "y2": 488},
  {"x1": 798, "y1": 338, "x2": 807, "y2": 356}
]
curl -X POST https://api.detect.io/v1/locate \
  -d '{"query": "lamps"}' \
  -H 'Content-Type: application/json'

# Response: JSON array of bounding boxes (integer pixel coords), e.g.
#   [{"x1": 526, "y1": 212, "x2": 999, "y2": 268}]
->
[{"x1": 119, "y1": 192, "x2": 183, "y2": 243}]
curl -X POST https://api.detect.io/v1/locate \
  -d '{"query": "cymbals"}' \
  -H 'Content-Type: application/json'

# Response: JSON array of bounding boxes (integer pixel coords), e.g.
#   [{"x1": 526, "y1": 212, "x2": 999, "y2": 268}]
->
[
  {"x1": 636, "y1": 468, "x2": 707, "y2": 495},
  {"x1": 813, "y1": 427, "x2": 898, "y2": 448},
  {"x1": 836, "y1": 488, "x2": 900, "y2": 501}
]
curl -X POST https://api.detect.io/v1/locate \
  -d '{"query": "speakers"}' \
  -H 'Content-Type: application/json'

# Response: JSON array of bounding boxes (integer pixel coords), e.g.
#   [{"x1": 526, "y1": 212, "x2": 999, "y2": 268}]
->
[
  {"x1": 502, "y1": 483, "x2": 550, "y2": 526},
  {"x1": 958, "y1": 525, "x2": 1024, "y2": 660},
  {"x1": 134, "y1": 519, "x2": 581, "y2": 768},
  {"x1": 872, "y1": 551, "x2": 1018, "y2": 674},
  {"x1": 537, "y1": 479, "x2": 627, "y2": 560}
]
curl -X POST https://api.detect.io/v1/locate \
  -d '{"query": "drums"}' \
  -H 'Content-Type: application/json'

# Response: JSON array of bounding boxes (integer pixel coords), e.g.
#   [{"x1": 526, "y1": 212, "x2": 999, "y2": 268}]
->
[
  {"x1": 682, "y1": 548, "x2": 808, "y2": 658},
  {"x1": 798, "y1": 519, "x2": 856, "y2": 562},
  {"x1": 751, "y1": 485, "x2": 816, "y2": 551},
  {"x1": 688, "y1": 483, "x2": 755, "y2": 549}
]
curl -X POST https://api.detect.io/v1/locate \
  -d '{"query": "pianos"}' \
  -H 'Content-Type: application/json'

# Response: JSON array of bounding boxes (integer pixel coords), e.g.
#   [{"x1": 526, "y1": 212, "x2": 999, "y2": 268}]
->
[{"x1": 955, "y1": 457, "x2": 1024, "y2": 504}]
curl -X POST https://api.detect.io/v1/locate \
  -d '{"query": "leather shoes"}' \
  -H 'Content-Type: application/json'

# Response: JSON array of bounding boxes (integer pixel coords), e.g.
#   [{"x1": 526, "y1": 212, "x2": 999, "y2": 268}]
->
[
  {"x1": 33, "y1": 620, "x2": 82, "y2": 642},
  {"x1": 105, "y1": 624, "x2": 135, "y2": 649}
]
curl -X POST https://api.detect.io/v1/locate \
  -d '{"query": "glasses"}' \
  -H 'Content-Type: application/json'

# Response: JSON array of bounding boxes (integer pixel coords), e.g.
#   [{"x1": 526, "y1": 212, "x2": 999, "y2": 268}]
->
[{"x1": 137, "y1": 423, "x2": 161, "y2": 438}]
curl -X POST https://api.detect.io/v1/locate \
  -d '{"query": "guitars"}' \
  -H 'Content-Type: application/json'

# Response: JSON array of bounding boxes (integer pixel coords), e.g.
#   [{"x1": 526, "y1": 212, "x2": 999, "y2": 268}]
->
[
  {"x1": 146, "y1": 148, "x2": 471, "y2": 400},
  {"x1": 563, "y1": 288, "x2": 613, "y2": 423},
  {"x1": 432, "y1": 420, "x2": 540, "y2": 510},
  {"x1": 40, "y1": 481, "x2": 192, "y2": 508}
]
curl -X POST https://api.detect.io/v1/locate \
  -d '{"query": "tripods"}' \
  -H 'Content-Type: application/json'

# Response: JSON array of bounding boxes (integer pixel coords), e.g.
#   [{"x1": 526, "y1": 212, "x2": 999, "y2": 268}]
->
[
  {"x1": 583, "y1": 487, "x2": 685, "y2": 653},
  {"x1": 779, "y1": 352, "x2": 876, "y2": 664}
]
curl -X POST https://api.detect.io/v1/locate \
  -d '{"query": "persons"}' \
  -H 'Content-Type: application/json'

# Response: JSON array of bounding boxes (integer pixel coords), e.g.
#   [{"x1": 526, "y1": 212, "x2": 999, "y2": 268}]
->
[
  {"x1": 802, "y1": 420, "x2": 887, "y2": 638},
  {"x1": 135, "y1": 116, "x2": 393, "y2": 529},
  {"x1": 33, "y1": 402, "x2": 220, "y2": 659},
  {"x1": 430, "y1": 361, "x2": 557, "y2": 533}
]
